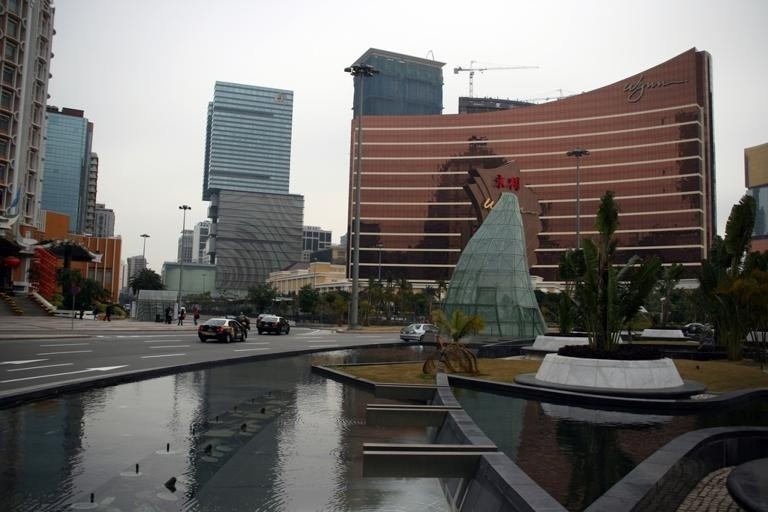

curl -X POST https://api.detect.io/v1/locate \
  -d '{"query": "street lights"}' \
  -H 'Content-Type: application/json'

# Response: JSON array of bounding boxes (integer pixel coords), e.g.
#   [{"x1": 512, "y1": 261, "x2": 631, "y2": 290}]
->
[
  {"x1": 375, "y1": 240, "x2": 384, "y2": 281},
  {"x1": 141, "y1": 234, "x2": 150, "y2": 256},
  {"x1": 567, "y1": 149, "x2": 591, "y2": 246},
  {"x1": 345, "y1": 62, "x2": 381, "y2": 329},
  {"x1": 179, "y1": 205, "x2": 191, "y2": 305}
]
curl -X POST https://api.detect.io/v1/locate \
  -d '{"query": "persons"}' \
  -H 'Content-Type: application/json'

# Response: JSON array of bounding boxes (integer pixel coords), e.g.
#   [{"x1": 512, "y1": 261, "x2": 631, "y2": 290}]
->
[
  {"x1": 193, "y1": 307, "x2": 199, "y2": 326},
  {"x1": 164, "y1": 306, "x2": 172, "y2": 324},
  {"x1": 92, "y1": 304, "x2": 100, "y2": 321},
  {"x1": 78, "y1": 302, "x2": 85, "y2": 320},
  {"x1": 103, "y1": 305, "x2": 113, "y2": 321},
  {"x1": 177, "y1": 308, "x2": 185, "y2": 326}
]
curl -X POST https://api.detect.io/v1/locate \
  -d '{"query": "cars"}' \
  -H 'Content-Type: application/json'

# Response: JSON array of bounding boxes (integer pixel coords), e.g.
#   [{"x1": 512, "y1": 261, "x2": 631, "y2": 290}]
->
[
  {"x1": 400, "y1": 324, "x2": 438, "y2": 343},
  {"x1": 256, "y1": 314, "x2": 290, "y2": 335},
  {"x1": 198, "y1": 318, "x2": 247, "y2": 343},
  {"x1": 682, "y1": 323, "x2": 714, "y2": 340}
]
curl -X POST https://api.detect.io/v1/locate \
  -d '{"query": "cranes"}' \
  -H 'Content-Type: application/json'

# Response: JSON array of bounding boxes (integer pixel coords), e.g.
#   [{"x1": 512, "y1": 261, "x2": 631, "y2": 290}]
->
[{"x1": 454, "y1": 61, "x2": 542, "y2": 97}]
